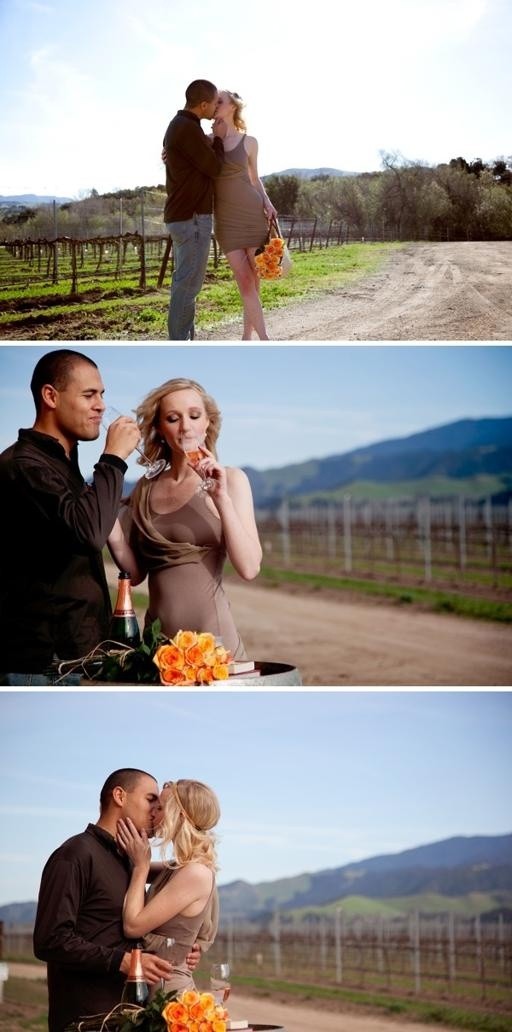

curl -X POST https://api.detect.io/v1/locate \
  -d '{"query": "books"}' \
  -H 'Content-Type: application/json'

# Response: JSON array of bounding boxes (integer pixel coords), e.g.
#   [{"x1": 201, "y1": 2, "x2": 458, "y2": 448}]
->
[
  {"x1": 223, "y1": 1017, "x2": 250, "y2": 1030},
  {"x1": 211, "y1": 661, "x2": 256, "y2": 675}
]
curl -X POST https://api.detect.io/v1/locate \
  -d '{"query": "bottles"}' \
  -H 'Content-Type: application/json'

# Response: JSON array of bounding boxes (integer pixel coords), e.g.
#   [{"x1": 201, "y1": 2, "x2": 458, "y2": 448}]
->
[
  {"x1": 120, "y1": 942, "x2": 151, "y2": 1013},
  {"x1": 106, "y1": 571, "x2": 141, "y2": 681}
]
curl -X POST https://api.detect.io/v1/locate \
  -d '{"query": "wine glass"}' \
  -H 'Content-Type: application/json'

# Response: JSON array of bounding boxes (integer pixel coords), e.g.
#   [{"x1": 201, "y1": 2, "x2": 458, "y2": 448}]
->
[
  {"x1": 210, "y1": 960, "x2": 231, "y2": 1004},
  {"x1": 97, "y1": 404, "x2": 168, "y2": 480},
  {"x1": 154, "y1": 936, "x2": 178, "y2": 993},
  {"x1": 181, "y1": 431, "x2": 216, "y2": 498}
]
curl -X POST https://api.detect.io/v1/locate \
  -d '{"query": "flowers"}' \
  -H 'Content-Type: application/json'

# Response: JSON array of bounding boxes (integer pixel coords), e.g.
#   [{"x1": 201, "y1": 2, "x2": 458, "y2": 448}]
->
[
  {"x1": 72, "y1": 988, "x2": 228, "y2": 1032},
  {"x1": 252, "y1": 237, "x2": 292, "y2": 282},
  {"x1": 50, "y1": 620, "x2": 229, "y2": 688}
]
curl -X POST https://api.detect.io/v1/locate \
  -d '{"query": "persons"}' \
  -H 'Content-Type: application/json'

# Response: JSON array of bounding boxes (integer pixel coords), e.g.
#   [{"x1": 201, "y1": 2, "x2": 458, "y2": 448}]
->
[
  {"x1": 2, "y1": 349, "x2": 145, "y2": 684},
  {"x1": 34, "y1": 768, "x2": 202, "y2": 1031},
  {"x1": 105, "y1": 375, "x2": 263, "y2": 660},
  {"x1": 161, "y1": 91, "x2": 279, "y2": 340},
  {"x1": 161, "y1": 80, "x2": 226, "y2": 339},
  {"x1": 115, "y1": 778, "x2": 221, "y2": 1001}
]
